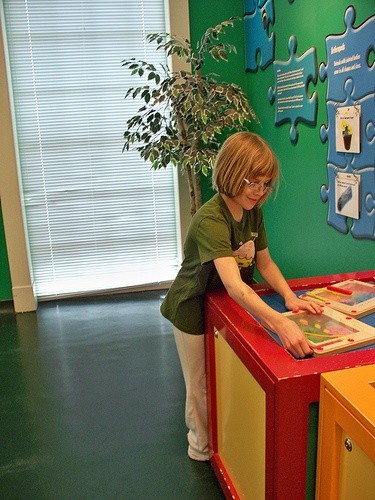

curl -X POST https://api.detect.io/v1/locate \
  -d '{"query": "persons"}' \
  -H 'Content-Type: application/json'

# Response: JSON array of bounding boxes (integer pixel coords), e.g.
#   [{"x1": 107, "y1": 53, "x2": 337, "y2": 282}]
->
[{"x1": 160, "y1": 132, "x2": 325, "y2": 461}]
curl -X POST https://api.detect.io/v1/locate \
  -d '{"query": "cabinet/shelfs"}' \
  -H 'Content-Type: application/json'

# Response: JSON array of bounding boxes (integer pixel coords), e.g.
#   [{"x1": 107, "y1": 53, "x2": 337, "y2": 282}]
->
[{"x1": 201, "y1": 270, "x2": 375, "y2": 500}]
